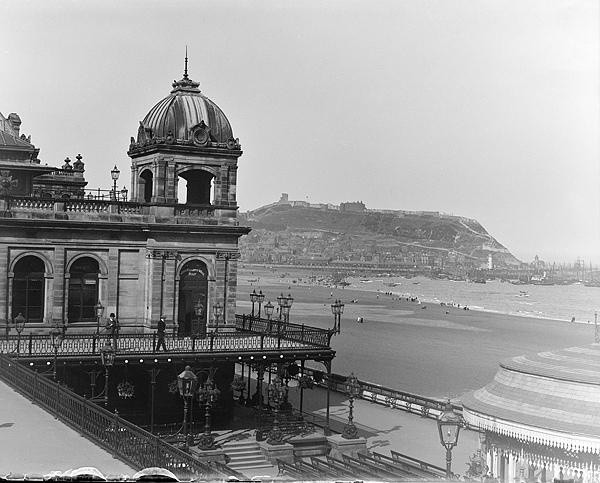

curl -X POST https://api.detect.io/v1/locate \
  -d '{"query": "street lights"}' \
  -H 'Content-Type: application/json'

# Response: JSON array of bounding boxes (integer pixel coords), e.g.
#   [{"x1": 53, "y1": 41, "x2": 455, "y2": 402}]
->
[
  {"x1": 249, "y1": 288, "x2": 257, "y2": 319},
  {"x1": 264, "y1": 301, "x2": 276, "y2": 334},
  {"x1": 211, "y1": 301, "x2": 223, "y2": 333},
  {"x1": 330, "y1": 299, "x2": 345, "y2": 332},
  {"x1": 257, "y1": 290, "x2": 265, "y2": 319},
  {"x1": 93, "y1": 301, "x2": 105, "y2": 336},
  {"x1": 100, "y1": 337, "x2": 117, "y2": 411},
  {"x1": 14, "y1": 311, "x2": 26, "y2": 360},
  {"x1": 435, "y1": 398, "x2": 465, "y2": 483},
  {"x1": 193, "y1": 298, "x2": 205, "y2": 336},
  {"x1": 175, "y1": 364, "x2": 197, "y2": 448},
  {"x1": 277, "y1": 291, "x2": 294, "y2": 324},
  {"x1": 49, "y1": 321, "x2": 64, "y2": 382},
  {"x1": 342, "y1": 370, "x2": 362, "y2": 439},
  {"x1": 195, "y1": 376, "x2": 219, "y2": 449},
  {"x1": 268, "y1": 377, "x2": 286, "y2": 446},
  {"x1": 110, "y1": 165, "x2": 121, "y2": 202}
]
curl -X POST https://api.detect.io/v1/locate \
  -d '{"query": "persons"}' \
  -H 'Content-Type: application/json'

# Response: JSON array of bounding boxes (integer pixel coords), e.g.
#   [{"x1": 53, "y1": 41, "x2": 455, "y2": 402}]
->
[
  {"x1": 105, "y1": 313, "x2": 117, "y2": 344},
  {"x1": 155, "y1": 314, "x2": 168, "y2": 352}
]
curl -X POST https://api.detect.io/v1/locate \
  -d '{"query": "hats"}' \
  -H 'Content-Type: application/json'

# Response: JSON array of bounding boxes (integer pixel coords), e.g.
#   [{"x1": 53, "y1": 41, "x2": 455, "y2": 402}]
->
[
  {"x1": 160, "y1": 314, "x2": 168, "y2": 318},
  {"x1": 109, "y1": 313, "x2": 116, "y2": 318}
]
